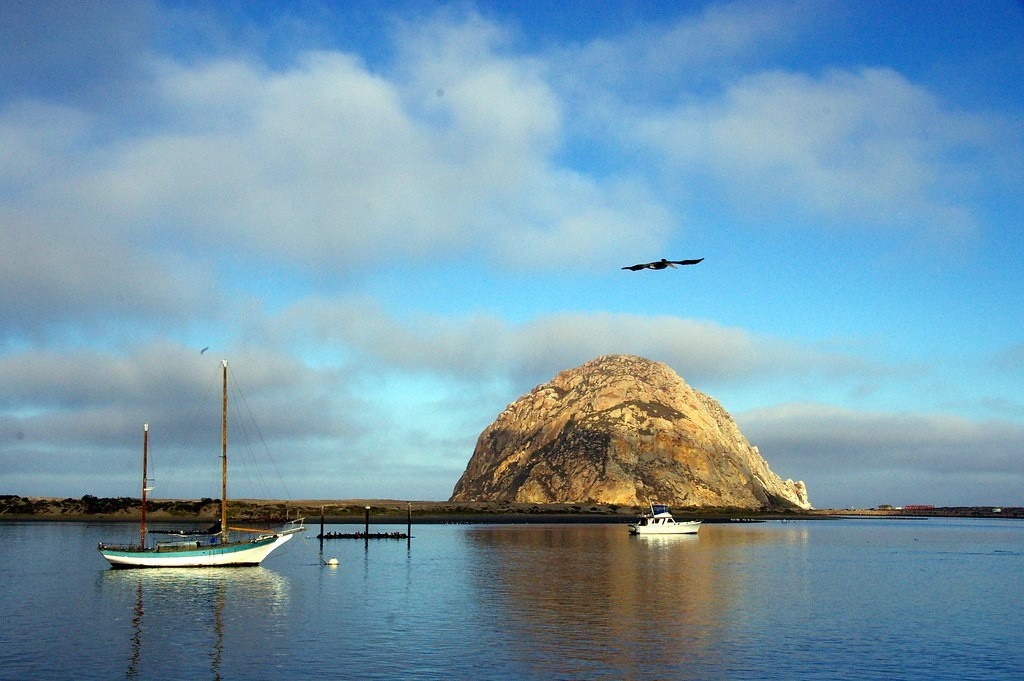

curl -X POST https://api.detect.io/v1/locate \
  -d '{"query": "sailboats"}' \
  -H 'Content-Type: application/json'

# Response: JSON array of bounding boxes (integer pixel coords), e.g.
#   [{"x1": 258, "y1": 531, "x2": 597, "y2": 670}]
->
[{"x1": 97, "y1": 356, "x2": 304, "y2": 570}]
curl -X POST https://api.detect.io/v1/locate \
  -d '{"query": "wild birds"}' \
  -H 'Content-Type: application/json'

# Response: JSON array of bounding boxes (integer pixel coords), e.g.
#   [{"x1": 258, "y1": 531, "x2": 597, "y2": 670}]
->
[{"x1": 622, "y1": 255, "x2": 706, "y2": 271}]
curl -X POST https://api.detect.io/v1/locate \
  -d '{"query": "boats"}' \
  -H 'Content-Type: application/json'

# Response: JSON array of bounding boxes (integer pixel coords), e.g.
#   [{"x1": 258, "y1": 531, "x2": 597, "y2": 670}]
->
[{"x1": 627, "y1": 497, "x2": 703, "y2": 536}]
